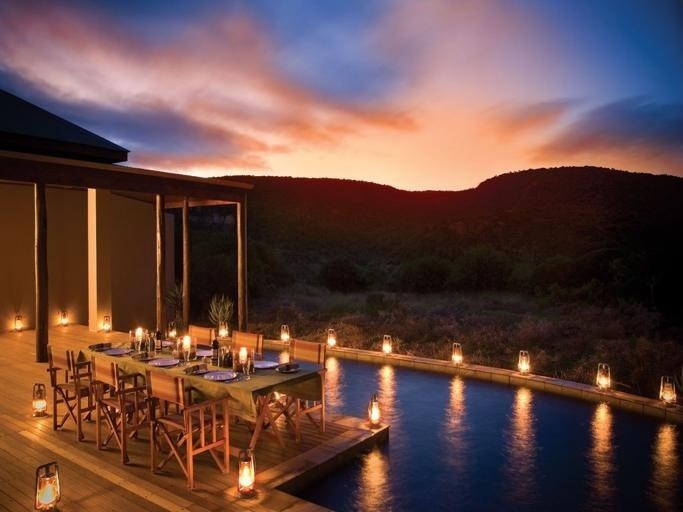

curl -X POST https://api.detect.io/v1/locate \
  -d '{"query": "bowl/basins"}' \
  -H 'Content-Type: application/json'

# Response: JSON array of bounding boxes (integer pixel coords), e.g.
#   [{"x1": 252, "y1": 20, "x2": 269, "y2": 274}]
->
[{"x1": 279, "y1": 363, "x2": 299, "y2": 374}]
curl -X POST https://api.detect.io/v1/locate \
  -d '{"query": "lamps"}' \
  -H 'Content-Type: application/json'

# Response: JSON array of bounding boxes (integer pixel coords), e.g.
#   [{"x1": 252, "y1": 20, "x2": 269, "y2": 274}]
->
[
  {"x1": 382, "y1": 334, "x2": 392, "y2": 354},
  {"x1": 34, "y1": 461, "x2": 61, "y2": 512},
  {"x1": 451, "y1": 342, "x2": 463, "y2": 365},
  {"x1": 326, "y1": 327, "x2": 337, "y2": 348},
  {"x1": 31, "y1": 382, "x2": 49, "y2": 418},
  {"x1": 595, "y1": 362, "x2": 611, "y2": 391},
  {"x1": 61, "y1": 310, "x2": 68, "y2": 327},
  {"x1": 367, "y1": 392, "x2": 382, "y2": 430},
  {"x1": 658, "y1": 375, "x2": 677, "y2": 408},
  {"x1": 237, "y1": 449, "x2": 257, "y2": 500},
  {"x1": 15, "y1": 315, "x2": 24, "y2": 332},
  {"x1": 103, "y1": 315, "x2": 110, "y2": 332},
  {"x1": 279, "y1": 321, "x2": 290, "y2": 342},
  {"x1": 517, "y1": 350, "x2": 530, "y2": 376},
  {"x1": 218, "y1": 318, "x2": 229, "y2": 338}
]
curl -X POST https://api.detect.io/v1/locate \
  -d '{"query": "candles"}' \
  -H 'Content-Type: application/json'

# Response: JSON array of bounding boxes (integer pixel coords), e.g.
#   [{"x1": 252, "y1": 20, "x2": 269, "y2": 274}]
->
[
  {"x1": 239, "y1": 347, "x2": 247, "y2": 364},
  {"x1": 183, "y1": 335, "x2": 190, "y2": 348},
  {"x1": 136, "y1": 328, "x2": 142, "y2": 338}
]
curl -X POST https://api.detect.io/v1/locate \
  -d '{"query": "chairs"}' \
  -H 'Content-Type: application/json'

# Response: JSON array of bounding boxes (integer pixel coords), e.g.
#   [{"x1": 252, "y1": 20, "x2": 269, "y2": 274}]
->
[
  {"x1": 260, "y1": 338, "x2": 327, "y2": 444},
  {"x1": 164, "y1": 324, "x2": 216, "y2": 416},
  {"x1": 90, "y1": 354, "x2": 168, "y2": 465},
  {"x1": 47, "y1": 343, "x2": 116, "y2": 442},
  {"x1": 145, "y1": 366, "x2": 232, "y2": 490},
  {"x1": 231, "y1": 329, "x2": 264, "y2": 428}
]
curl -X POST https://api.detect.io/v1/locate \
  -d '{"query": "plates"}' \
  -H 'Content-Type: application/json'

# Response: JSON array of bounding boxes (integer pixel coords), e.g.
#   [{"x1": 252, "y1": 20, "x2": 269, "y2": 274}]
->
[
  {"x1": 148, "y1": 358, "x2": 178, "y2": 367},
  {"x1": 87, "y1": 342, "x2": 113, "y2": 351},
  {"x1": 206, "y1": 370, "x2": 235, "y2": 382},
  {"x1": 253, "y1": 360, "x2": 278, "y2": 370},
  {"x1": 104, "y1": 348, "x2": 130, "y2": 355}
]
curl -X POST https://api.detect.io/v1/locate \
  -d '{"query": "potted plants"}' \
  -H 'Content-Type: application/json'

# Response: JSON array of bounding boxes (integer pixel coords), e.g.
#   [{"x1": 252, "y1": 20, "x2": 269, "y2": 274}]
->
[{"x1": 392, "y1": 336, "x2": 400, "y2": 351}]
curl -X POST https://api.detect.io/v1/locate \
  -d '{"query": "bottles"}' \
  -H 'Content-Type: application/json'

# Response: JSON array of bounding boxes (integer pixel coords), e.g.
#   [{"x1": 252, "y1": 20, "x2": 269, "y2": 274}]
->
[
  {"x1": 175, "y1": 334, "x2": 256, "y2": 374},
  {"x1": 126, "y1": 328, "x2": 161, "y2": 361}
]
